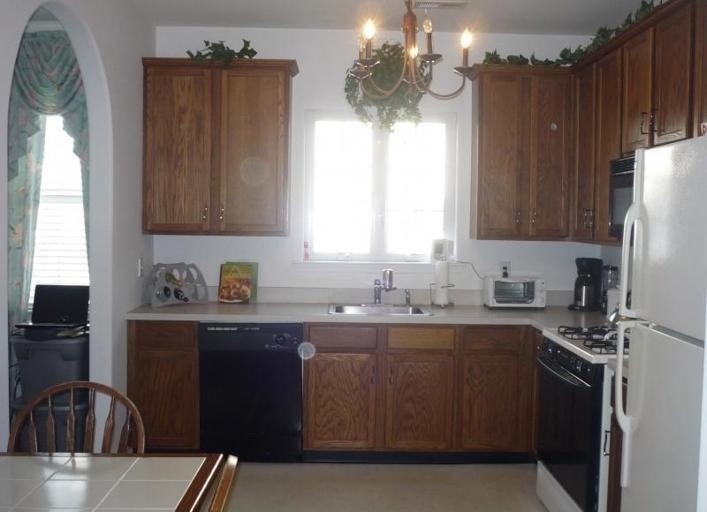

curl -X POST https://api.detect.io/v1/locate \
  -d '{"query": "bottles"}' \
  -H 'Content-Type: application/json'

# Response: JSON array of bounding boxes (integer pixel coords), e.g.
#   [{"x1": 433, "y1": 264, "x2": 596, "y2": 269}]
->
[
  {"x1": 164, "y1": 287, "x2": 189, "y2": 302},
  {"x1": 165, "y1": 272, "x2": 183, "y2": 287}
]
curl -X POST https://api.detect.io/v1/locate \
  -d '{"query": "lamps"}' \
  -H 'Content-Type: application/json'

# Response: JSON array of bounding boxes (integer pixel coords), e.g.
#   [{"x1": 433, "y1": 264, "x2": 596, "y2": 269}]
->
[{"x1": 348, "y1": 1, "x2": 473, "y2": 101}]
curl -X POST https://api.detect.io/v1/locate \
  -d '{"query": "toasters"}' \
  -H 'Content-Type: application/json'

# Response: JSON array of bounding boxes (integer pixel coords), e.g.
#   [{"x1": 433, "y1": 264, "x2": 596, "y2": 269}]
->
[{"x1": 485, "y1": 276, "x2": 547, "y2": 308}]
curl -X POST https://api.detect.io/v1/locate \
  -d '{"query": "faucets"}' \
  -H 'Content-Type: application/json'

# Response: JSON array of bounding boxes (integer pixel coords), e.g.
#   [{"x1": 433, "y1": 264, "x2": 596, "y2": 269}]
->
[{"x1": 373, "y1": 268, "x2": 397, "y2": 303}]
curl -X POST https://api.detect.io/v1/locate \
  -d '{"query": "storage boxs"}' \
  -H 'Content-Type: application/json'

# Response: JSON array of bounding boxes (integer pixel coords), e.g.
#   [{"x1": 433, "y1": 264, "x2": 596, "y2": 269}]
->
[
  {"x1": 10, "y1": 333, "x2": 88, "y2": 405},
  {"x1": 10, "y1": 396, "x2": 88, "y2": 453}
]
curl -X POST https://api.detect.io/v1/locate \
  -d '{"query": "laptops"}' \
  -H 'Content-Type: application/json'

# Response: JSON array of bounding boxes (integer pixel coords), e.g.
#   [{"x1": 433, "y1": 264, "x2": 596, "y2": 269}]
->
[{"x1": 14, "y1": 283, "x2": 90, "y2": 329}]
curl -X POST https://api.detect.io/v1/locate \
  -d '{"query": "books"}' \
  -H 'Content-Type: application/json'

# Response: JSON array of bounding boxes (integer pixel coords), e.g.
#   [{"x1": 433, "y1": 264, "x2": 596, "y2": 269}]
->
[{"x1": 216, "y1": 261, "x2": 259, "y2": 304}]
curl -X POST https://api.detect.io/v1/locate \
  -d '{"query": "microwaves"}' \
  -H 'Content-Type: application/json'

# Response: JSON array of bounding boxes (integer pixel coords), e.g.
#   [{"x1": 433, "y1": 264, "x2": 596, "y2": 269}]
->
[
  {"x1": 608, "y1": 158, "x2": 642, "y2": 242},
  {"x1": 568, "y1": 255, "x2": 604, "y2": 308}
]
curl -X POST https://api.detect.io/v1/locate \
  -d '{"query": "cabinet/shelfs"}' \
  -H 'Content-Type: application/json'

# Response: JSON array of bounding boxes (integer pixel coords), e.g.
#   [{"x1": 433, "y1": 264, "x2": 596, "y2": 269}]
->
[
  {"x1": 457, "y1": 323, "x2": 544, "y2": 464},
  {"x1": 467, "y1": 63, "x2": 572, "y2": 242},
  {"x1": 572, "y1": 33, "x2": 622, "y2": 245},
  {"x1": 126, "y1": 319, "x2": 201, "y2": 452},
  {"x1": 301, "y1": 322, "x2": 460, "y2": 463},
  {"x1": 141, "y1": 56, "x2": 300, "y2": 236},
  {"x1": 622, "y1": 0, "x2": 694, "y2": 155}
]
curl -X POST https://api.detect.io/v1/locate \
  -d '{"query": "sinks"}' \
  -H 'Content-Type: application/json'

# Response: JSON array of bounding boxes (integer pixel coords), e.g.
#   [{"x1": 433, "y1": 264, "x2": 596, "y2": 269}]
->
[{"x1": 328, "y1": 302, "x2": 433, "y2": 316}]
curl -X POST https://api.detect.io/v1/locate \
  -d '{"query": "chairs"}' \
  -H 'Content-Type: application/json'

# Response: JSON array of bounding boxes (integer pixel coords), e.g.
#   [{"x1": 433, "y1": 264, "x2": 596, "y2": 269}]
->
[
  {"x1": 6, "y1": 379, "x2": 146, "y2": 454},
  {"x1": 205, "y1": 453, "x2": 240, "y2": 512}
]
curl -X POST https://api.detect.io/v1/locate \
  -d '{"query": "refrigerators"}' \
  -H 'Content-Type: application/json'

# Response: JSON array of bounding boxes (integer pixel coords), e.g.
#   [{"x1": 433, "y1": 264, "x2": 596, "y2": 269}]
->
[{"x1": 615, "y1": 135, "x2": 705, "y2": 507}]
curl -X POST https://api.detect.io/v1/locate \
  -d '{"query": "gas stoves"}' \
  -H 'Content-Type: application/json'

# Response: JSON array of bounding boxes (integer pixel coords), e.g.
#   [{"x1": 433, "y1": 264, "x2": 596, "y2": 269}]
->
[{"x1": 537, "y1": 327, "x2": 630, "y2": 432}]
